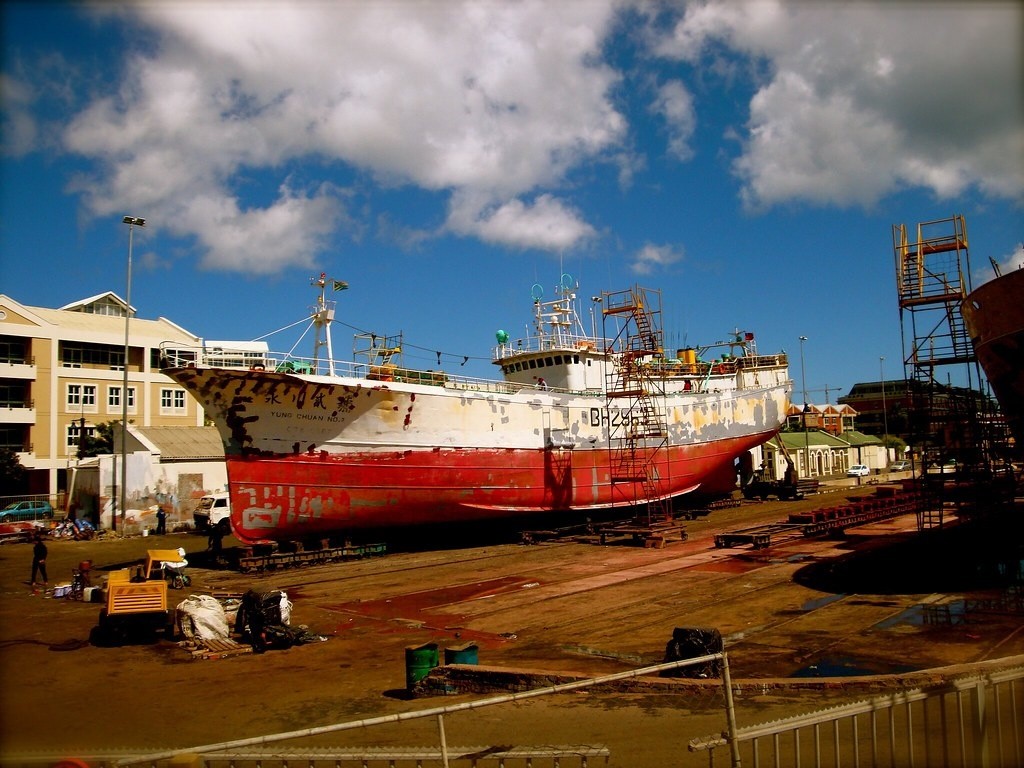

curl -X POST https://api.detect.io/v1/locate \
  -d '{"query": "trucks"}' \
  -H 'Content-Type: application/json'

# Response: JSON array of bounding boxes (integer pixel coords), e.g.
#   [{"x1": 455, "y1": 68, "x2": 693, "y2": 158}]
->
[{"x1": 193, "y1": 492, "x2": 231, "y2": 534}]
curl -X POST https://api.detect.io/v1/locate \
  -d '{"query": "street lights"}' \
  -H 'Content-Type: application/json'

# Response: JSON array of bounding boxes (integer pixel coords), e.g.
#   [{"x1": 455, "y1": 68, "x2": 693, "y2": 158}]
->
[
  {"x1": 798, "y1": 335, "x2": 809, "y2": 476},
  {"x1": 881, "y1": 358, "x2": 892, "y2": 469},
  {"x1": 121, "y1": 213, "x2": 148, "y2": 521}
]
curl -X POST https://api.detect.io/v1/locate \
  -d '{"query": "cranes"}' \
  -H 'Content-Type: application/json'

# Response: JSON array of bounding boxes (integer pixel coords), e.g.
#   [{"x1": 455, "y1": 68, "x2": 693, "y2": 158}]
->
[{"x1": 793, "y1": 385, "x2": 844, "y2": 435}]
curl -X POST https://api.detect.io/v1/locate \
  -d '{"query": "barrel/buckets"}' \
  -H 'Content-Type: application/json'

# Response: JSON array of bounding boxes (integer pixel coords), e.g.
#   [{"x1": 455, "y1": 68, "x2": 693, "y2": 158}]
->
[
  {"x1": 445, "y1": 641, "x2": 478, "y2": 665},
  {"x1": 143, "y1": 529, "x2": 148, "y2": 537},
  {"x1": 406, "y1": 639, "x2": 439, "y2": 697},
  {"x1": 54, "y1": 582, "x2": 82, "y2": 599}
]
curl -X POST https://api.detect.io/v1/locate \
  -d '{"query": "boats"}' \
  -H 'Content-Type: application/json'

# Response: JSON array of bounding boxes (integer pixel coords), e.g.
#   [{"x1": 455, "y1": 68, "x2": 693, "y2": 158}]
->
[{"x1": 156, "y1": 259, "x2": 794, "y2": 549}]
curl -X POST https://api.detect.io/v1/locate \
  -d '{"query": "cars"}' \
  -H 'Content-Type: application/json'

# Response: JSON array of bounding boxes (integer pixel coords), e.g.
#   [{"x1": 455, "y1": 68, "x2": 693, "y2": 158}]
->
[
  {"x1": 890, "y1": 461, "x2": 911, "y2": 472},
  {"x1": 847, "y1": 465, "x2": 871, "y2": 477},
  {"x1": 0, "y1": 501, "x2": 55, "y2": 522}
]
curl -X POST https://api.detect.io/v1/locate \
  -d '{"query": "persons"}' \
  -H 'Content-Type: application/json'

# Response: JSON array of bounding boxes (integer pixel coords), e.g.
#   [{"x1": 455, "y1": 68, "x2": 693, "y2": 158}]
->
[
  {"x1": 684, "y1": 380, "x2": 691, "y2": 390},
  {"x1": 31, "y1": 536, "x2": 49, "y2": 586},
  {"x1": 156, "y1": 507, "x2": 166, "y2": 534},
  {"x1": 538, "y1": 378, "x2": 547, "y2": 391},
  {"x1": 209, "y1": 524, "x2": 225, "y2": 551}
]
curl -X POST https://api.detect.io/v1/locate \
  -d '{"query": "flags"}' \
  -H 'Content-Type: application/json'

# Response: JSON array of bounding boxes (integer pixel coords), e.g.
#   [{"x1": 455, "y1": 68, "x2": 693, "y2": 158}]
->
[{"x1": 333, "y1": 281, "x2": 349, "y2": 291}]
